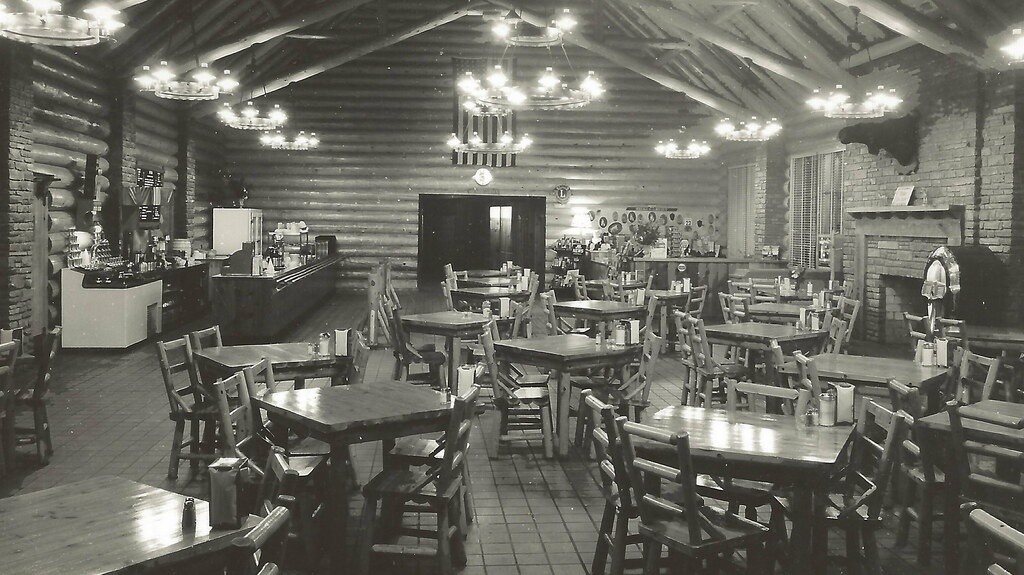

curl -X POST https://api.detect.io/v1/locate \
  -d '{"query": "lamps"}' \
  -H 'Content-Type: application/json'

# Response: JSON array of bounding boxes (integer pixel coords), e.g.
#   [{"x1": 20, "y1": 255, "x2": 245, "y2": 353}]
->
[
  {"x1": 491, "y1": 0, "x2": 577, "y2": 45},
  {"x1": 653, "y1": 124, "x2": 712, "y2": 160},
  {"x1": 805, "y1": 4, "x2": 905, "y2": 121},
  {"x1": 711, "y1": 57, "x2": 784, "y2": 143},
  {"x1": 0, "y1": 0, "x2": 126, "y2": 47},
  {"x1": 461, "y1": 96, "x2": 513, "y2": 117},
  {"x1": 454, "y1": 45, "x2": 604, "y2": 112},
  {"x1": 133, "y1": 0, "x2": 241, "y2": 101},
  {"x1": 446, "y1": 117, "x2": 533, "y2": 154},
  {"x1": 259, "y1": 130, "x2": 321, "y2": 151},
  {"x1": 216, "y1": 46, "x2": 289, "y2": 130}
]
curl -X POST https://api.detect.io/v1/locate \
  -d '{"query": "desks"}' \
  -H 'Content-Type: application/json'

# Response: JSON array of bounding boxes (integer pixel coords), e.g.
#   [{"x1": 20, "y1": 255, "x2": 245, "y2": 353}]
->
[
  {"x1": 190, "y1": 343, "x2": 352, "y2": 395},
  {"x1": 492, "y1": 331, "x2": 640, "y2": 461},
  {"x1": 755, "y1": 289, "x2": 820, "y2": 302},
  {"x1": 400, "y1": 311, "x2": 517, "y2": 395},
  {"x1": 583, "y1": 279, "x2": 646, "y2": 299},
  {"x1": 735, "y1": 301, "x2": 842, "y2": 326},
  {"x1": 251, "y1": 379, "x2": 457, "y2": 574},
  {"x1": 467, "y1": 270, "x2": 505, "y2": 276},
  {"x1": 449, "y1": 286, "x2": 530, "y2": 314},
  {"x1": 917, "y1": 399, "x2": 1024, "y2": 565},
  {"x1": 932, "y1": 325, "x2": 1023, "y2": 352},
  {"x1": 546, "y1": 300, "x2": 646, "y2": 339},
  {"x1": 1, "y1": 474, "x2": 265, "y2": 575},
  {"x1": 611, "y1": 288, "x2": 698, "y2": 354},
  {"x1": 614, "y1": 404, "x2": 857, "y2": 575},
  {"x1": 691, "y1": 322, "x2": 829, "y2": 415},
  {"x1": 457, "y1": 277, "x2": 521, "y2": 287},
  {"x1": 778, "y1": 352, "x2": 950, "y2": 415}
]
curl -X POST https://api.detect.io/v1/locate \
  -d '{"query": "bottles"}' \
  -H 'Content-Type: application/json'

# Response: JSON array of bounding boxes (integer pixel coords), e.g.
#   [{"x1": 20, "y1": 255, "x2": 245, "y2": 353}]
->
[
  {"x1": 922, "y1": 342, "x2": 934, "y2": 366},
  {"x1": 812, "y1": 313, "x2": 819, "y2": 330},
  {"x1": 320, "y1": 333, "x2": 330, "y2": 355},
  {"x1": 934, "y1": 354, "x2": 937, "y2": 365},
  {"x1": 813, "y1": 408, "x2": 818, "y2": 425},
  {"x1": 819, "y1": 393, "x2": 834, "y2": 427},
  {"x1": 440, "y1": 388, "x2": 447, "y2": 404},
  {"x1": 615, "y1": 325, "x2": 625, "y2": 346},
  {"x1": 447, "y1": 388, "x2": 451, "y2": 403},
  {"x1": 805, "y1": 409, "x2": 812, "y2": 426},
  {"x1": 812, "y1": 293, "x2": 818, "y2": 306},
  {"x1": 308, "y1": 342, "x2": 312, "y2": 354},
  {"x1": 314, "y1": 343, "x2": 319, "y2": 354}
]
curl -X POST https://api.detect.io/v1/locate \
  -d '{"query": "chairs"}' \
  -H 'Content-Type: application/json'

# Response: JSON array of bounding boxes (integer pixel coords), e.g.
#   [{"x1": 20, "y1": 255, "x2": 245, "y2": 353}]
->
[{"x1": 0, "y1": 262, "x2": 1024, "y2": 575}]
